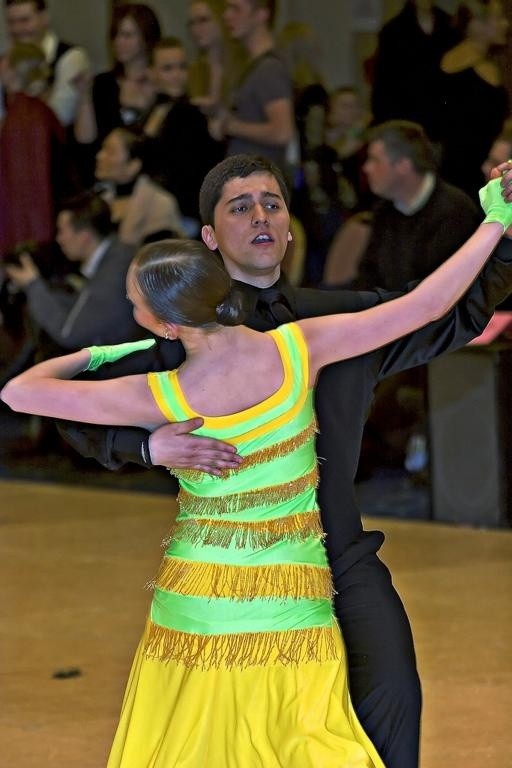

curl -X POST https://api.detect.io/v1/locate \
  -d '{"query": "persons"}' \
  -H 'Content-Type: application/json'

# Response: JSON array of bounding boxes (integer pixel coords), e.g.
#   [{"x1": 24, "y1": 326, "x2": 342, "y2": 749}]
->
[
  {"x1": 0, "y1": 2, "x2": 510, "y2": 517},
  {"x1": 51, "y1": 154, "x2": 511, "y2": 767},
  {"x1": 0, "y1": 176, "x2": 510, "y2": 768}
]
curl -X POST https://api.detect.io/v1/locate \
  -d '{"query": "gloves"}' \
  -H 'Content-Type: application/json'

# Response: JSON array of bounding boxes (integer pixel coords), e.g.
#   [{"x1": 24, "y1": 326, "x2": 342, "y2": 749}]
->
[
  {"x1": 81, "y1": 337, "x2": 154, "y2": 371},
  {"x1": 478, "y1": 177, "x2": 512, "y2": 234}
]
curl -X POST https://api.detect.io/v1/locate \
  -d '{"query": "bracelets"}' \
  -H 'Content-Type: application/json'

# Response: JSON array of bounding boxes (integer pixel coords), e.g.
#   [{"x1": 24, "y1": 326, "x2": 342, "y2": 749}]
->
[{"x1": 141, "y1": 434, "x2": 153, "y2": 468}]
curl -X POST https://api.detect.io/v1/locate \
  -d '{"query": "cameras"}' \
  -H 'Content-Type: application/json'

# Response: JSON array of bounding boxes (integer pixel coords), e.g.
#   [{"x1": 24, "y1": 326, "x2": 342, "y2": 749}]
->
[{"x1": 3, "y1": 241, "x2": 37, "y2": 266}]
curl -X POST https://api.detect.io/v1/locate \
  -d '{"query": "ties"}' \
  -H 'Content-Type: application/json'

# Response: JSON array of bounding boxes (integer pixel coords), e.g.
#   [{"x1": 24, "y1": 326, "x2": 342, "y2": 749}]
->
[{"x1": 257, "y1": 288, "x2": 294, "y2": 322}]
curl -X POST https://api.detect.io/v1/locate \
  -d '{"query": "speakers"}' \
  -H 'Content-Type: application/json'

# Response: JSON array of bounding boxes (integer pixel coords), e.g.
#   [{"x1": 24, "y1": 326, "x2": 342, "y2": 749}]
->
[{"x1": 428, "y1": 338, "x2": 512, "y2": 528}]
